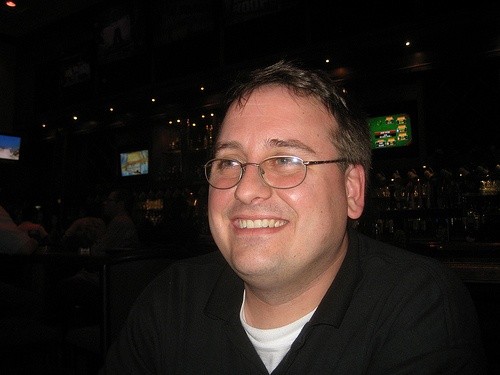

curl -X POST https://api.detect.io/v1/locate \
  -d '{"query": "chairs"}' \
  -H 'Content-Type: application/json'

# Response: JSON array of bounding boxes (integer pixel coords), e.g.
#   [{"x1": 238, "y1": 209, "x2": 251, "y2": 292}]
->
[{"x1": 56, "y1": 243, "x2": 193, "y2": 365}]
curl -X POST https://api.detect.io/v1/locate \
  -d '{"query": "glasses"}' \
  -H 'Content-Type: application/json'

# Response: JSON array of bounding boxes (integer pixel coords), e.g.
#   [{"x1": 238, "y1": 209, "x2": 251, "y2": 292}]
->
[{"x1": 204, "y1": 157, "x2": 353, "y2": 190}]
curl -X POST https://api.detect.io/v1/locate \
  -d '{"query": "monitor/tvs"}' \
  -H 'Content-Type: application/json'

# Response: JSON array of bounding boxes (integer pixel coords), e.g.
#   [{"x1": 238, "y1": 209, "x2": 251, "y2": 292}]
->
[
  {"x1": 365, "y1": 113, "x2": 412, "y2": 150},
  {"x1": 120, "y1": 149, "x2": 149, "y2": 176},
  {"x1": 0, "y1": 134, "x2": 21, "y2": 161}
]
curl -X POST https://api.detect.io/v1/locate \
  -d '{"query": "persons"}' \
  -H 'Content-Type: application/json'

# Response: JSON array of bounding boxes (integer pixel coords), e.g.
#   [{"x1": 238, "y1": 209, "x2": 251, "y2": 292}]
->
[
  {"x1": 0, "y1": 191, "x2": 139, "y2": 329},
  {"x1": 95, "y1": 61, "x2": 500, "y2": 375}
]
